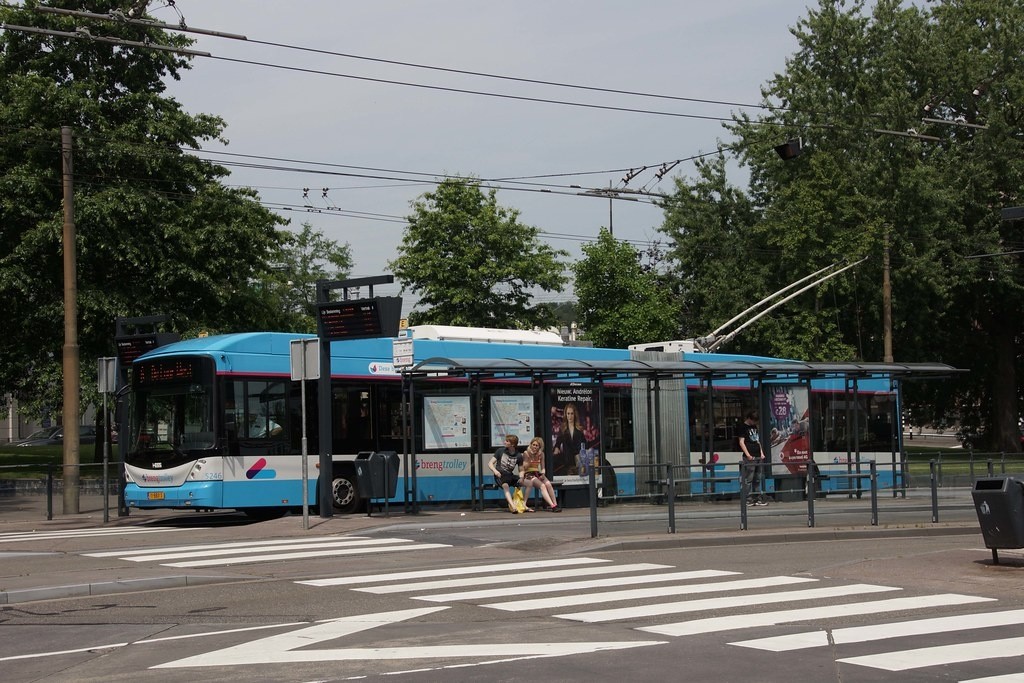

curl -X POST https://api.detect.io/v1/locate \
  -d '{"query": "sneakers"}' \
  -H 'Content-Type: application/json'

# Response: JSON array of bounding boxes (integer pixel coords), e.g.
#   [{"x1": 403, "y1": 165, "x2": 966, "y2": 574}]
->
[
  {"x1": 754, "y1": 498, "x2": 768, "y2": 506},
  {"x1": 556, "y1": 505, "x2": 562, "y2": 511},
  {"x1": 553, "y1": 506, "x2": 559, "y2": 511},
  {"x1": 745, "y1": 499, "x2": 755, "y2": 506}
]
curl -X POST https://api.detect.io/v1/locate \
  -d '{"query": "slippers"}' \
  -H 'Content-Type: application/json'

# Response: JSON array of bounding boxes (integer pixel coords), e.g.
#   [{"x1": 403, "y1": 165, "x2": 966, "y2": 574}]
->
[
  {"x1": 512, "y1": 508, "x2": 518, "y2": 513},
  {"x1": 525, "y1": 508, "x2": 535, "y2": 512}
]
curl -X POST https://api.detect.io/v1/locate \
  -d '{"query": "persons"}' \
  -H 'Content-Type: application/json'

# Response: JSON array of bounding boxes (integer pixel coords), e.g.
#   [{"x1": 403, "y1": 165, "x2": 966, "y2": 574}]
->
[
  {"x1": 770, "y1": 428, "x2": 784, "y2": 446},
  {"x1": 787, "y1": 419, "x2": 809, "y2": 436},
  {"x1": 553, "y1": 403, "x2": 587, "y2": 475},
  {"x1": 737, "y1": 409, "x2": 769, "y2": 506},
  {"x1": 340, "y1": 394, "x2": 412, "y2": 439},
  {"x1": 237, "y1": 402, "x2": 282, "y2": 439},
  {"x1": 488, "y1": 435, "x2": 534, "y2": 513},
  {"x1": 522, "y1": 437, "x2": 562, "y2": 512}
]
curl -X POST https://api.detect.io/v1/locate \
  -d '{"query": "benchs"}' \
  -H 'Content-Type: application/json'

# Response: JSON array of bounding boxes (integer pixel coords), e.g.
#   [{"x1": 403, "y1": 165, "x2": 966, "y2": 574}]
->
[
  {"x1": 813, "y1": 472, "x2": 880, "y2": 500},
  {"x1": 644, "y1": 476, "x2": 740, "y2": 504},
  {"x1": 473, "y1": 481, "x2": 564, "y2": 513}
]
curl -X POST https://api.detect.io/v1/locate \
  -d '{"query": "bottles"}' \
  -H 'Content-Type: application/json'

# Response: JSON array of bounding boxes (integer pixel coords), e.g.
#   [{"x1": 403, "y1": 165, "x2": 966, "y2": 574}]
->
[{"x1": 578, "y1": 441, "x2": 600, "y2": 477}]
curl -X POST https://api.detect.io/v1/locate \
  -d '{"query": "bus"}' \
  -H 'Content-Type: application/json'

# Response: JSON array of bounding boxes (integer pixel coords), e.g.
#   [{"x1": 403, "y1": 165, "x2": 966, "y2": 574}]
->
[{"x1": 115, "y1": 273, "x2": 907, "y2": 519}]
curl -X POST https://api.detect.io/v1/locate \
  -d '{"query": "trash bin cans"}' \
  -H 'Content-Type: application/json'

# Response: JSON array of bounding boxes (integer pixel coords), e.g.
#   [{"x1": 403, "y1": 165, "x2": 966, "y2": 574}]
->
[
  {"x1": 970, "y1": 473, "x2": 1024, "y2": 565},
  {"x1": 354, "y1": 450, "x2": 400, "y2": 518}
]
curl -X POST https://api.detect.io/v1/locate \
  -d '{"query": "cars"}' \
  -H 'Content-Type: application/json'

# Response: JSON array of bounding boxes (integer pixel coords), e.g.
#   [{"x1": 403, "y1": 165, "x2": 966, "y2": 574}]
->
[{"x1": 6, "y1": 425, "x2": 104, "y2": 447}]
